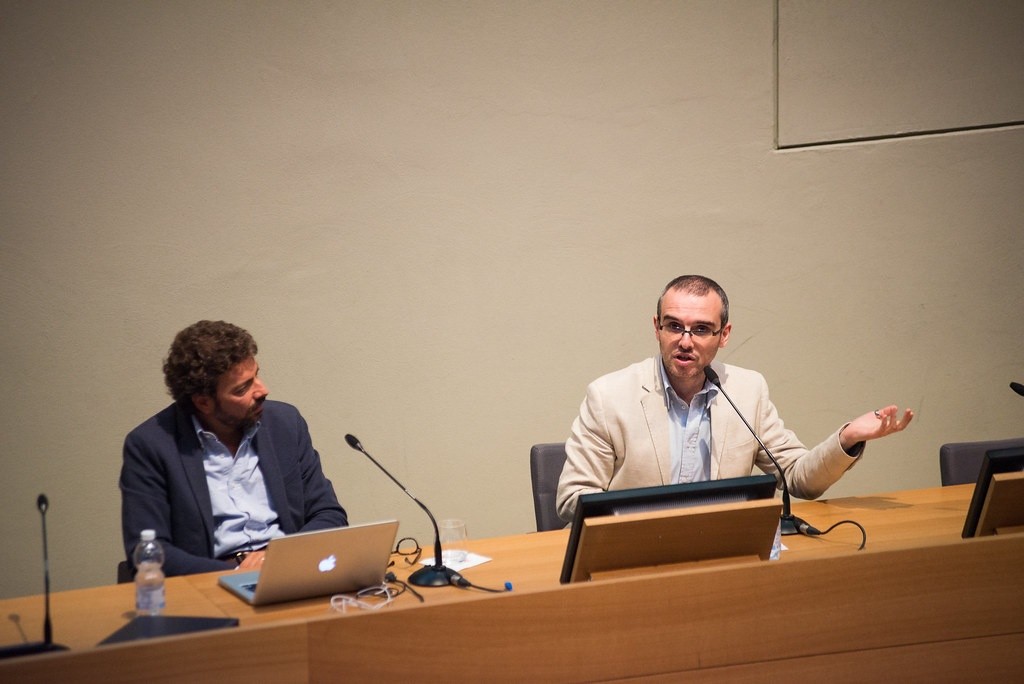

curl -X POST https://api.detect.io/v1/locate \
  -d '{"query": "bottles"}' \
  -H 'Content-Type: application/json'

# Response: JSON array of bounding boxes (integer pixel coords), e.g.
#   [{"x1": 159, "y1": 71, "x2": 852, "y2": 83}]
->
[{"x1": 134, "y1": 529, "x2": 165, "y2": 618}]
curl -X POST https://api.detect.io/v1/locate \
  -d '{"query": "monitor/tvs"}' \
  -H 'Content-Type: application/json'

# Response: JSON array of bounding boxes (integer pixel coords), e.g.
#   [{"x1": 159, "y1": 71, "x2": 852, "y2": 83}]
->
[
  {"x1": 962, "y1": 447, "x2": 1024, "y2": 539},
  {"x1": 560, "y1": 473, "x2": 777, "y2": 586}
]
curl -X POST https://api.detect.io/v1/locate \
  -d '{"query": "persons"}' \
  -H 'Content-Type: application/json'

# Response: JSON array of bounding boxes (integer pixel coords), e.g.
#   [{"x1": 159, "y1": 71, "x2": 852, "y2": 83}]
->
[
  {"x1": 556, "y1": 275, "x2": 913, "y2": 531},
  {"x1": 118, "y1": 320, "x2": 351, "y2": 582}
]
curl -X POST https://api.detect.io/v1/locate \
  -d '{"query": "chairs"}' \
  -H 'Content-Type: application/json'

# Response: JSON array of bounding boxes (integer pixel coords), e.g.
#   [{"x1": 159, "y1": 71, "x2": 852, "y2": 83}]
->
[
  {"x1": 531, "y1": 443, "x2": 567, "y2": 531},
  {"x1": 939, "y1": 438, "x2": 1024, "y2": 487}
]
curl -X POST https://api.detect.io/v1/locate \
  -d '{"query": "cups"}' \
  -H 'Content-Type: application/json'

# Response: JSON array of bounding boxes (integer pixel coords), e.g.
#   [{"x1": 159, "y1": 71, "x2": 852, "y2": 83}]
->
[
  {"x1": 431, "y1": 519, "x2": 469, "y2": 563},
  {"x1": 769, "y1": 519, "x2": 781, "y2": 560}
]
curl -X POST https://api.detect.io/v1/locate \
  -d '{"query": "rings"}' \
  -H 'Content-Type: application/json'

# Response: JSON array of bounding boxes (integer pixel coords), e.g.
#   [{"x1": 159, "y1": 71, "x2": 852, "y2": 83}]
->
[
  {"x1": 874, "y1": 410, "x2": 881, "y2": 419},
  {"x1": 261, "y1": 557, "x2": 265, "y2": 560}
]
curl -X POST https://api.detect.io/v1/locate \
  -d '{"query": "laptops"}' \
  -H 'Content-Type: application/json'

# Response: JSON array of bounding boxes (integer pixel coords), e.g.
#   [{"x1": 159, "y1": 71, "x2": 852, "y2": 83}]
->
[{"x1": 217, "y1": 520, "x2": 401, "y2": 607}]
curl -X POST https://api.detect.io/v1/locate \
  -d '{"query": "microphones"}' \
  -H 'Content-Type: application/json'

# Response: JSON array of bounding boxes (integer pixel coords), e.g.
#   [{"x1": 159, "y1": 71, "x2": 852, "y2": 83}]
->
[
  {"x1": 0, "y1": 494, "x2": 69, "y2": 659},
  {"x1": 1010, "y1": 382, "x2": 1024, "y2": 397},
  {"x1": 703, "y1": 365, "x2": 810, "y2": 535},
  {"x1": 345, "y1": 433, "x2": 463, "y2": 588},
  {"x1": 792, "y1": 516, "x2": 821, "y2": 536}
]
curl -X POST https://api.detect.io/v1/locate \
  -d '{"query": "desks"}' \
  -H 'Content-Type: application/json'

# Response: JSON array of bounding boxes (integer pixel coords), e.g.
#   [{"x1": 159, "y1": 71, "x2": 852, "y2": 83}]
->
[{"x1": 0, "y1": 483, "x2": 1024, "y2": 684}]
